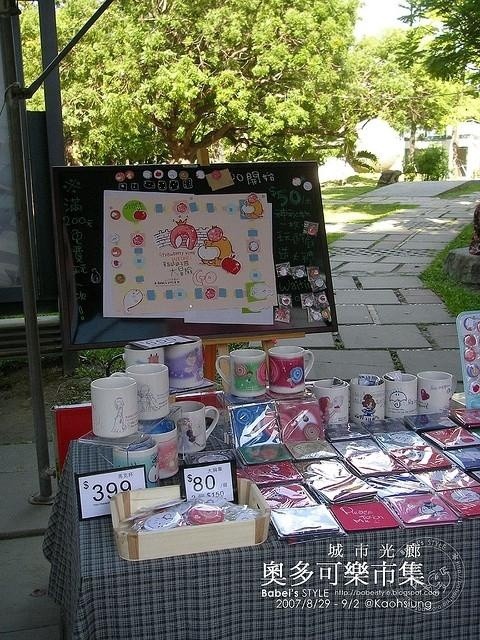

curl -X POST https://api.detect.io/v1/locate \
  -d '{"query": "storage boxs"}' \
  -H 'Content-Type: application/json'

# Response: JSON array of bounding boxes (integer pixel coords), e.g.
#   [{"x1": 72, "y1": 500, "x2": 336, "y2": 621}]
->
[{"x1": 109, "y1": 477, "x2": 271, "y2": 561}]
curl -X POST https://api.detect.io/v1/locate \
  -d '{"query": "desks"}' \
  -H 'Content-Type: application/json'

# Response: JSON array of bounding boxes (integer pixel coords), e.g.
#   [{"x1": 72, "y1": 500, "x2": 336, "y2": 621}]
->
[{"x1": 42, "y1": 425, "x2": 480, "y2": 640}]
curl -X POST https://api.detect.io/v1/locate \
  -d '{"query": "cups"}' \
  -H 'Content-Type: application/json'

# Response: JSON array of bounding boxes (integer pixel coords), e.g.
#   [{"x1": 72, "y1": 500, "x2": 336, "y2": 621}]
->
[
  {"x1": 313, "y1": 371, "x2": 454, "y2": 425},
  {"x1": 215, "y1": 344, "x2": 314, "y2": 399},
  {"x1": 89, "y1": 337, "x2": 221, "y2": 490}
]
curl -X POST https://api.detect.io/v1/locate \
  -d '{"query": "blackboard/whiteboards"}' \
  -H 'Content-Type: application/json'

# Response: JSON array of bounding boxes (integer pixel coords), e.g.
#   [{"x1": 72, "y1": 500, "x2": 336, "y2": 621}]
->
[{"x1": 50, "y1": 161, "x2": 338, "y2": 351}]
